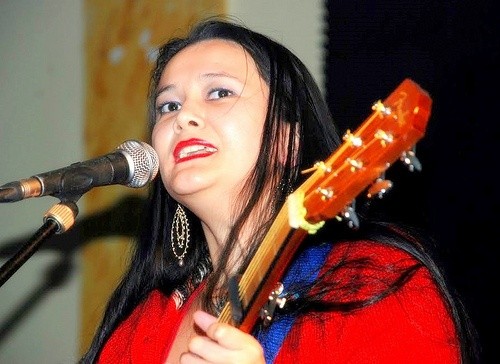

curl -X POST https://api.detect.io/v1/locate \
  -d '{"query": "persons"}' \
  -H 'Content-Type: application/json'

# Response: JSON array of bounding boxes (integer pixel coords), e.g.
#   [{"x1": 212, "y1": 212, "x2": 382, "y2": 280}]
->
[{"x1": 78, "y1": 13, "x2": 483, "y2": 364}]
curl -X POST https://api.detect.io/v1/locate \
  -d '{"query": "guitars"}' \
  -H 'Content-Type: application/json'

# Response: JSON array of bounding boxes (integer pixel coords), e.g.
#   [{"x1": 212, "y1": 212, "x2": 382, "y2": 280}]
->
[{"x1": 216, "y1": 77, "x2": 434, "y2": 335}]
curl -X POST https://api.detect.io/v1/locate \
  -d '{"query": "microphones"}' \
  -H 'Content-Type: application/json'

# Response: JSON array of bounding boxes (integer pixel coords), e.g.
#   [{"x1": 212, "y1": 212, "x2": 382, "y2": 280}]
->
[{"x1": 0, "y1": 139, "x2": 160, "y2": 203}]
